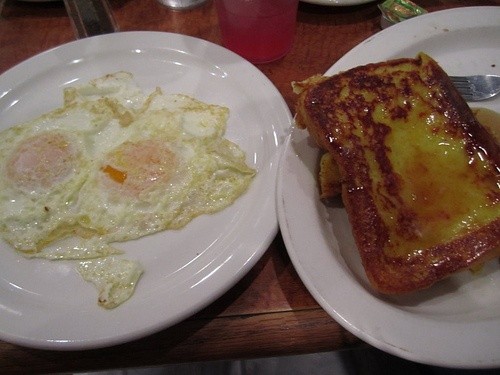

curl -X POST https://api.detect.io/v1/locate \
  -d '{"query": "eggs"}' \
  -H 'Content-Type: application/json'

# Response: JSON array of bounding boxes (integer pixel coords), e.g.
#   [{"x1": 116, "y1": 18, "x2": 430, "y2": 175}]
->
[
  {"x1": 0, "y1": 71, "x2": 146, "y2": 257},
  {"x1": 64, "y1": 87, "x2": 256, "y2": 239}
]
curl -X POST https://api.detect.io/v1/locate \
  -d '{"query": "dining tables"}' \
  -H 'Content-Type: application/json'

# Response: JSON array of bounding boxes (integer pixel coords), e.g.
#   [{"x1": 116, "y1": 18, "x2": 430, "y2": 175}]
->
[{"x1": 1, "y1": 1, "x2": 485, "y2": 372}]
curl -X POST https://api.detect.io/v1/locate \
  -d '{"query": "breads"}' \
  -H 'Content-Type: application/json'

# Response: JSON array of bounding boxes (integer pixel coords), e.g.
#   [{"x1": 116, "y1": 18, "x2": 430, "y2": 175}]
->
[{"x1": 295, "y1": 54, "x2": 500, "y2": 293}]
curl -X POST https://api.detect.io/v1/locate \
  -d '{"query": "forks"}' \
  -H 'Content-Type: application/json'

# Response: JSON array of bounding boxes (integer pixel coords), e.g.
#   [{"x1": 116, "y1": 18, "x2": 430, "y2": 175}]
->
[{"x1": 445, "y1": 74, "x2": 500, "y2": 102}]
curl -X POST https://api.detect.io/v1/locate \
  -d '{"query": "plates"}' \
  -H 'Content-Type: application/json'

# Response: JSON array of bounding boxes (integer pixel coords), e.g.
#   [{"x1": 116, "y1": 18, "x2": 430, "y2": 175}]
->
[
  {"x1": 276, "y1": 6, "x2": 500, "y2": 370},
  {"x1": 0, "y1": 31, "x2": 295, "y2": 351}
]
